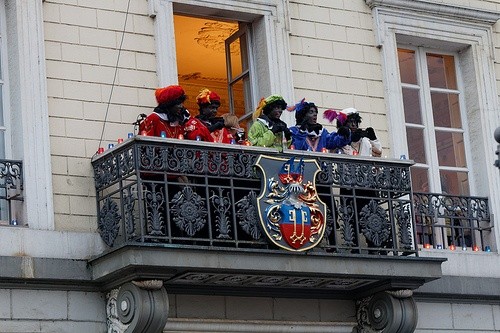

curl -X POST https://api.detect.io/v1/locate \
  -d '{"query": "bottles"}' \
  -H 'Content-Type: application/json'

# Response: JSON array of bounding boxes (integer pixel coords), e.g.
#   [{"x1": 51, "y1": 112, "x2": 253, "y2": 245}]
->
[
  {"x1": 358, "y1": 137, "x2": 372, "y2": 157},
  {"x1": 281, "y1": 131, "x2": 288, "y2": 149}
]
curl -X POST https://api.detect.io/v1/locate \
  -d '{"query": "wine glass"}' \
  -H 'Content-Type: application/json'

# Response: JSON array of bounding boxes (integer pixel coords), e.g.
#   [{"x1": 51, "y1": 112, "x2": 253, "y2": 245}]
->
[{"x1": 236, "y1": 127, "x2": 244, "y2": 143}]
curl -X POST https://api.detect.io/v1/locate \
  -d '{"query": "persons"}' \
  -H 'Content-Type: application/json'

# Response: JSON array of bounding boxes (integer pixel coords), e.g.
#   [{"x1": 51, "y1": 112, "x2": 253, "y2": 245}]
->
[
  {"x1": 331, "y1": 107, "x2": 382, "y2": 254},
  {"x1": 185, "y1": 87, "x2": 253, "y2": 175},
  {"x1": 287, "y1": 97, "x2": 352, "y2": 254},
  {"x1": 247, "y1": 95, "x2": 295, "y2": 149},
  {"x1": 139, "y1": 85, "x2": 189, "y2": 185}
]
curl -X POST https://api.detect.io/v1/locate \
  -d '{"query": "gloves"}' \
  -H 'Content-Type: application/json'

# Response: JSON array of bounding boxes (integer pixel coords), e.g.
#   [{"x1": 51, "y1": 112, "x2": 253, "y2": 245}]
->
[
  {"x1": 271, "y1": 123, "x2": 285, "y2": 133},
  {"x1": 338, "y1": 127, "x2": 350, "y2": 137},
  {"x1": 284, "y1": 128, "x2": 293, "y2": 141},
  {"x1": 351, "y1": 129, "x2": 362, "y2": 142},
  {"x1": 366, "y1": 127, "x2": 377, "y2": 140}
]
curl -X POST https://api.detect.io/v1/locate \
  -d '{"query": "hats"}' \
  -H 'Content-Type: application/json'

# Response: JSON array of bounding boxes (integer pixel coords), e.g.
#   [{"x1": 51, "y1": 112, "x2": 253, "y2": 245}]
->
[
  {"x1": 196, "y1": 89, "x2": 220, "y2": 107},
  {"x1": 288, "y1": 98, "x2": 318, "y2": 117},
  {"x1": 324, "y1": 107, "x2": 362, "y2": 123},
  {"x1": 155, "y1": 85, "x2": 186, "y2": 108},
  {"x1": 262, "y1": 95, "x2": 287, "y2": 113}
]
